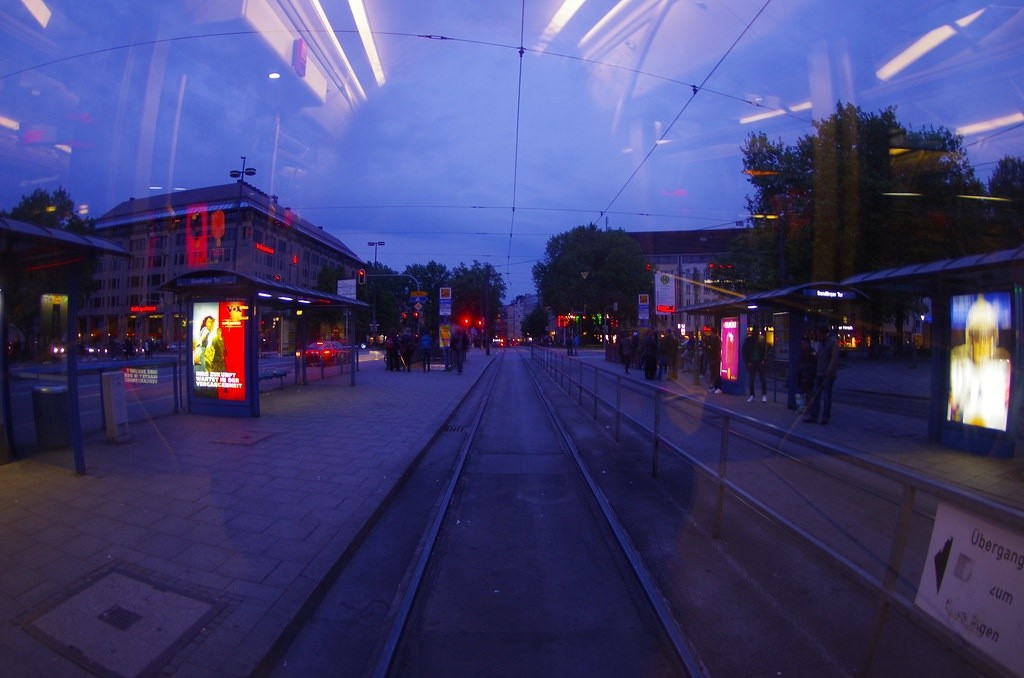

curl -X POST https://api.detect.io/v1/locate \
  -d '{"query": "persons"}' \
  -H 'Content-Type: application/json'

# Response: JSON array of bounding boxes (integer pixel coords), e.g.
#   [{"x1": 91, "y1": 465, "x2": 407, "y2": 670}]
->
[
  {"x1": 565, "y1": 333, "x2": 579, "y2": 357},
  {"x1": 742, "y1": 325, "x2": 768, "y2": 403},
  {"x1": 797, "y1": 325, "x2": 839, "y2": 424},
  {"x1": 947, "y1": 294, "x2": 1011, "y2": 432},
  {"x1": 194, "y1": 316, "x2": 228, "y2": 393},
  {"x1": 620, "y1": 327, "x2": 721, "y2": 402},
  {"x1": 386, "y1": 325, "x2": 468, "y2": 375}
]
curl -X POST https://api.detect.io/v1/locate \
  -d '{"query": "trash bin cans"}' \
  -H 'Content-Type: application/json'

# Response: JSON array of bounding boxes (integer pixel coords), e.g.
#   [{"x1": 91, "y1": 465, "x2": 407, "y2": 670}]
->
[{"x1": 33, "y1": 385, "x2": 71, "y2": 451}]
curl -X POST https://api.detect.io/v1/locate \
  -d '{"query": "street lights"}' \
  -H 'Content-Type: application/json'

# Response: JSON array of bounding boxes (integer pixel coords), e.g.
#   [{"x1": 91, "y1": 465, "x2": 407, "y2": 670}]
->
[
  {"x1": 229, "y1": 154, "x2": 255, "y2": 270},
  {"x1": 368, "y1": 241, "x2": 385, "y2": 269}
]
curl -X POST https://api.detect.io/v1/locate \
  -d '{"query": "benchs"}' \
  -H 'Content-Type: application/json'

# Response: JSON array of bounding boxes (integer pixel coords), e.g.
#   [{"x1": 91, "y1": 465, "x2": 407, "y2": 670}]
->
[{"x1": 259, "y1": 368, "x2": 295, "y2": 392}]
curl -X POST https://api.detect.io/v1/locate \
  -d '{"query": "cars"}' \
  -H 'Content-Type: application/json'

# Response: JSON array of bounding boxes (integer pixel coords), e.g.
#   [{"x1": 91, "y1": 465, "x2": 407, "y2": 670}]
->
[{"x1": 295, "y1": 338, "x2": 342, "y2": 364}]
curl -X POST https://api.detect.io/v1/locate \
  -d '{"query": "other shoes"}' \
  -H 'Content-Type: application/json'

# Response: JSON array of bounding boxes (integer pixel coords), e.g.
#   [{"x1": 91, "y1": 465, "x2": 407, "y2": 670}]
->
[
  {"x1": 803, "y1": 417, "x2": 819, "y2": 423},
  {"x1": 819, "y1": 417, "x2": 830, "y2": 425},
  {"x1": 709, "y1": 388, "x2": 715, "y2": 392},
  {"x1": 762, "y1": 395, "x2": 768, "y2": 403},
  {"x1": 715, "y1": 389, "x2": 722, "y2": 394},
  {"x1": 747, "y1": 396, "x2": 755, "y2": 403}
]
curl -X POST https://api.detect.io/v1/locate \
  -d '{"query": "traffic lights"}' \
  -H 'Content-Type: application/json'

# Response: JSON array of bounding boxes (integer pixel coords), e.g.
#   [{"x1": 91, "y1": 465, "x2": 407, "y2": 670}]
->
[
  {"x1": 292, "y1": 255, "x2": 299, "y2": 265},
  {"x1": 358, "y1": 268, "x2": 367, "y2": 284},
  {"x1": 275, "y1": 273, "x2": 282, "y2": 282}
]
